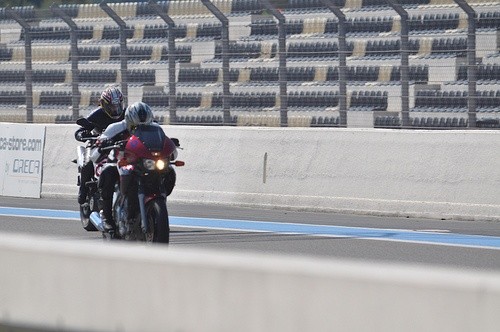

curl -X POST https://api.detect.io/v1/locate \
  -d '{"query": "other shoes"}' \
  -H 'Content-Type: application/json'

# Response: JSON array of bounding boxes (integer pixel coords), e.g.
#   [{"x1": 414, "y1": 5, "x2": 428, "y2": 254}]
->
[
  {"x1": 78, "y1": 193, "x2": 86, "y2": 204},
  {"x1": 102, "y1": 217, "x2": 115, "y2": 229}
]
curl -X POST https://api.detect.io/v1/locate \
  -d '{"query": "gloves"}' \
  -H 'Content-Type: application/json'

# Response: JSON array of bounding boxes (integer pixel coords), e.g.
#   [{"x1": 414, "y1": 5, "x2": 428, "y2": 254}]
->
[
  {"x1": 101, "y1": 138, "x2": 112, "y2": 147},
  {"x1": 171, "y1": 138, "x2": 180, "y2": 146},
  {"x1": 78, "y1": 130, "x2": 91, "y2": 142}
]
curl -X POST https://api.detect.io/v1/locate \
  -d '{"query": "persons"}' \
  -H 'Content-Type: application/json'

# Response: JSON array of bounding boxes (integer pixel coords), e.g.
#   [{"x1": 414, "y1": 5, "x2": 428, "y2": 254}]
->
[
  {"x1": 74, "y1": 88, "x2": 158, "y2": 204},
  {"x1": 90, "y1": 102, "x2": 180, "y2": 233}
]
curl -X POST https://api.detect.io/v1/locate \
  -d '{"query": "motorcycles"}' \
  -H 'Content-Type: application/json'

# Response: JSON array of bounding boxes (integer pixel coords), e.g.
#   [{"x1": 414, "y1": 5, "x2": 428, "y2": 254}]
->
[
  {"x1": 71, "y1": 117, "x2": 120, "y2": 232},
  {"x1": 85, "y1": 124, "x2": 185, "y2": 245}
]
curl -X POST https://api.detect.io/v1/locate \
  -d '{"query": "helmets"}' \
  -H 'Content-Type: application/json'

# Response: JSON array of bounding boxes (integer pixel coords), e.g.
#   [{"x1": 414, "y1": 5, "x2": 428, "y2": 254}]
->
[
  {"x1": 125, "y1": 102, "x2": 154, "y2": 133},
  {"x1": 99, "y1": 86, "x2": 125, "y2": 119}
]
